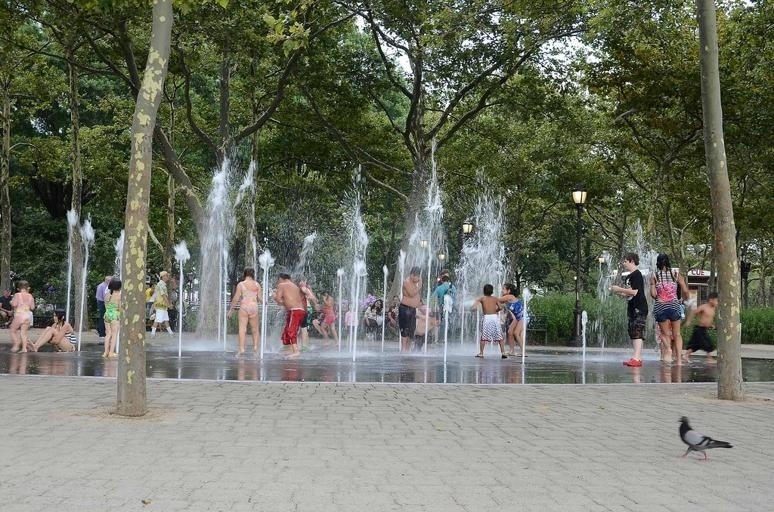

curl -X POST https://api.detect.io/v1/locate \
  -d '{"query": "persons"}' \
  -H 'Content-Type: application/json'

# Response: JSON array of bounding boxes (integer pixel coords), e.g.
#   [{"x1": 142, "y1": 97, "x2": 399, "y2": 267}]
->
[
  {"x1": 469, "y1": 283, "x2": 508, "y2": 358},
  {"x1": 97, "y1": 270, "x2": 177, "y2": 358},
  {"x1": 226, "y1": 267, "x2": 267, "y2": 355},
  {"x1": 0, "y1": 288, "x2": 14, "y2": 326},
  {"x1": 9, "y1": 279, "x2": 35, "y2": 353},
  {"x1": 497, "y1": 283, "x2": 530, "y2": 357},
  {"x1": 272, "y1": 265, "x2": 457, "y2": 358},
  {"x1": 27, "y1": 307, "x2": 77, "y2": 353},
  {"x1": 610, "y1": 253, "x2": 648, "y2": 366},
  {"x1": 651, "y1": 253, "x2": 689, "y2": 365},
  {"x1": 681, "y1": 290, "x2": 718, "y2": 363}
]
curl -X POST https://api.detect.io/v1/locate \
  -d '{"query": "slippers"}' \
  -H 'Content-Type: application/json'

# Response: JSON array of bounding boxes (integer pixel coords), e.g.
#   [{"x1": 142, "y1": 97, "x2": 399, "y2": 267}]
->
[
  {"x1": 516, "y1": 354, "x2": 528, "y2": 357},
  {"x1": 505, "y1": 352, "x2": 515, "y2": 356},
  {"x1": 475, "y1": 354, "x2": 483, "y2": 357},
  {"x1": 502, "y1": 354, "x2": 507, "y2": 358}
]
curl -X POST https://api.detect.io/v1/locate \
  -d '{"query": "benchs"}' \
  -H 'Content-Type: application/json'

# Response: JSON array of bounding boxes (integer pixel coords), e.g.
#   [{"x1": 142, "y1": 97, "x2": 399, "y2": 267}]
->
[
  {"x1": 471, "y1": 312, "x2": 549, "y2": 346},
  {"x1": 358, "y1": 307, "x2": 439, "y2": 342}
]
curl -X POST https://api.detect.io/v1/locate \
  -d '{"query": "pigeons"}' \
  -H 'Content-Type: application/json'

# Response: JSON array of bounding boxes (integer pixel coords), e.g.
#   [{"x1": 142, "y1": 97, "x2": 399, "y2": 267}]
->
[{"x1": 678, "y1": 416, "x2": 734, "y2": 461}]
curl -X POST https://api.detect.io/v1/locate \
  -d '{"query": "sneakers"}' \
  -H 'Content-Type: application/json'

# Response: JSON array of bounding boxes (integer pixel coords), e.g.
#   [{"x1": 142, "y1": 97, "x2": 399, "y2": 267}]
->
[
  {"x1": 624, "y1": 358, "x2": 633, "y2": 365},
  {"x1": 627, "y1": 359, "x2": 642, "y2": 367}
]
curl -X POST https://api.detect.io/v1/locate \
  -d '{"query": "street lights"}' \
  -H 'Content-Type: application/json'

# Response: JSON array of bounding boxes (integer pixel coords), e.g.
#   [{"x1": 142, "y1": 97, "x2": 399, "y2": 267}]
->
[
  {"x1": 417, "y1": 236, "x2": 429, "y2": 297},
  {"x1": 187, "y1": 273, "x2": 195, "y2": 304},
  {"x1": 437, "y1": 250, "x2": 444, "y2": 271},
  {"x1": 459, "y1": 219, "x2": 472, "y2": 305},
  {"x1": 571, "y1": 185, "x2": 589, "y2": 347},
  {"x1": 597, "y1": 255, "x2": 605, "y2": 299}
]
